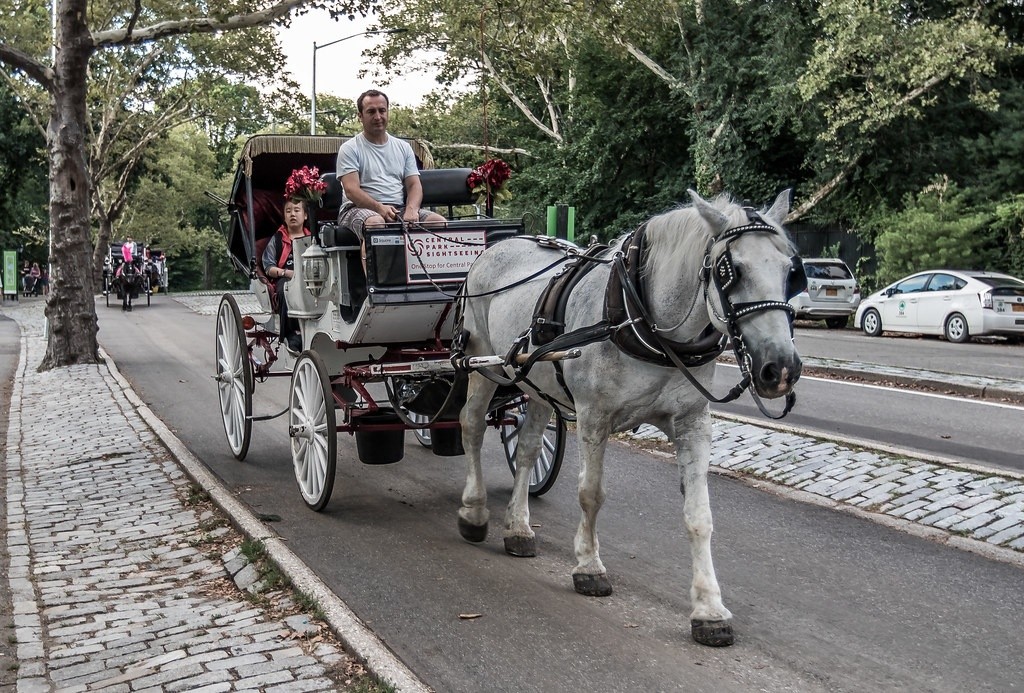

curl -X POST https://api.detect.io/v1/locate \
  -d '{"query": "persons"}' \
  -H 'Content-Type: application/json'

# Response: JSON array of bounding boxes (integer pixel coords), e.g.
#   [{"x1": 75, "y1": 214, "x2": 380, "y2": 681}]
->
[
  {"x1": 262, "y1": 197, "x2": 311, "y2": 354},
  {"x1": 336, "y1": 89, "x2": 449, "y2": 277},
  {"x1": 19, "y1": 236, "x2": 165, "y2": 300}
]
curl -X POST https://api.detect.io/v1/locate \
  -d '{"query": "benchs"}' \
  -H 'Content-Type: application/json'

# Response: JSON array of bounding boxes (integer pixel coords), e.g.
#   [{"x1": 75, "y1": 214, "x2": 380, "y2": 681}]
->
[
  {"x1": 318, "y1": 167, "x2": 494, "y2": 251},
  {"x1": 255, "y1": 228, "x2": 320, "y2": 334}
]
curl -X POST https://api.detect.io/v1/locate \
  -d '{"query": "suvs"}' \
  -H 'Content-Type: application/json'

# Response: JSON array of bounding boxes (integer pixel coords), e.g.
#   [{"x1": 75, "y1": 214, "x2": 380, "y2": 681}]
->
[{"x1": 788, "y1": 259, "x2": 860, "y2": 328}]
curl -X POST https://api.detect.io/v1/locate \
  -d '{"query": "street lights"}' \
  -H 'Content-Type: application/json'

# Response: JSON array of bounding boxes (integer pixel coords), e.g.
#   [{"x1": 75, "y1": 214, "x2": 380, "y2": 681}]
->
[
  {"x1": 273, "y1": 110, "x2": 339, "y2": 134},
  {"x1": 312, "y1": 29, "x2": 408, "y2": 135}
]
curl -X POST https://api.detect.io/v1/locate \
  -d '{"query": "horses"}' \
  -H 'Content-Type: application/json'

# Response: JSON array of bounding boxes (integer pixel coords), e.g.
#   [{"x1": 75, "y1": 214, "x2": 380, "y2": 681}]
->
[
  {"x1": 118, "y1": 258, "x2": 138, "y2": 312},
  {"x1": 458, "y1": 187, "x2": 802, "y2": 648}
]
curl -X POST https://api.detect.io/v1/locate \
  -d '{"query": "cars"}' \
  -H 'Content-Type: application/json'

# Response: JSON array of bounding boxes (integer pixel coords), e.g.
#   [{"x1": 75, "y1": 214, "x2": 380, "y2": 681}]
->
[{"x1": 854, "y1": 270, "x2": 1024, "y2": 344}]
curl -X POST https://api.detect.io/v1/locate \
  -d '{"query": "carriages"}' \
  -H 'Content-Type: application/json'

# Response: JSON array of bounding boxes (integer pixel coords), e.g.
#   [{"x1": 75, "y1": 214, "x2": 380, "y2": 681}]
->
[
  {"x1": 145, "y1": 249, "x2": 169, "y2": 296},
  {"x1": 106, "y1": 243, "x2": 151, "y2": 310},
  {"x1": 213, "y1": 134, "x2": 808, "y2": 647}
]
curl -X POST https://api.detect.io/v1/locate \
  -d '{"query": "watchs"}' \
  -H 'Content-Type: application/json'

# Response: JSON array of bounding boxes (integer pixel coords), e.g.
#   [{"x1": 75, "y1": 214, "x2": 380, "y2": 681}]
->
[{"x1": 282, "y1": 268, "x2": 288, "y2": 277}]
